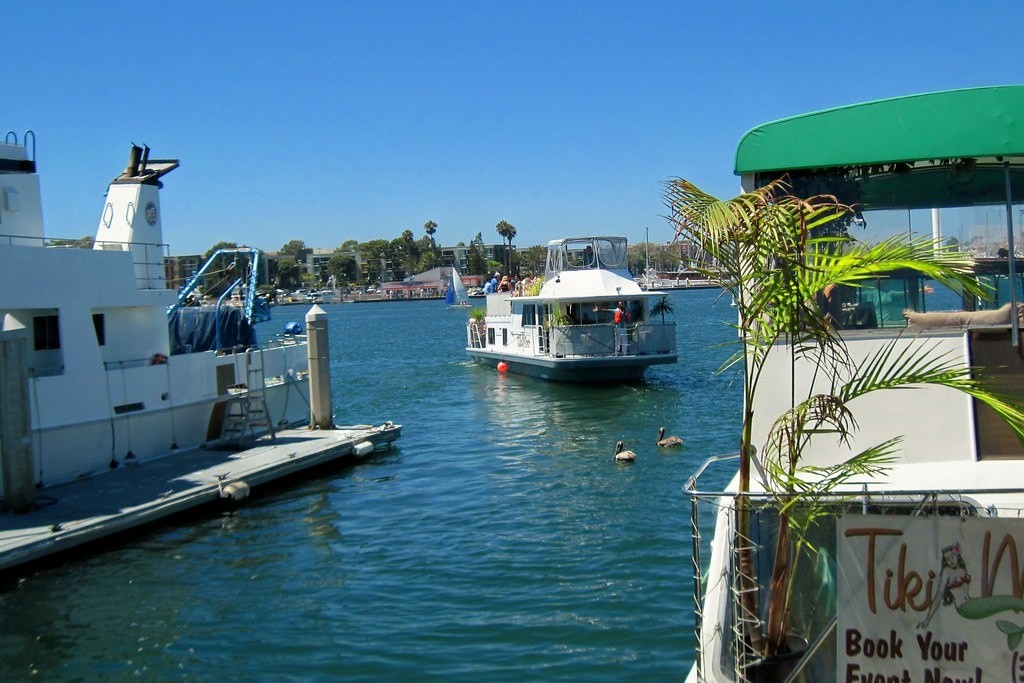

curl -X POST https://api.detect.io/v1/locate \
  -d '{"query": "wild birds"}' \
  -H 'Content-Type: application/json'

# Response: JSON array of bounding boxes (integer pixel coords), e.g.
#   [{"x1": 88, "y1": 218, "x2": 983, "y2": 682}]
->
[
  {"x1": 611, "y1": 440, "x2": 637, "y2": 464},
  {"x1": 655, "y1": 427, "x2": 684, "y2": 449}
]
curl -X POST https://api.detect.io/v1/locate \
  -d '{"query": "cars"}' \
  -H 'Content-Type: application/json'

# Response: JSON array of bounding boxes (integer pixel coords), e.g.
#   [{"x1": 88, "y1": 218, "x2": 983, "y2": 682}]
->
[{"x1": 293, "y1": 282, "x2": 380, "y2": 297}]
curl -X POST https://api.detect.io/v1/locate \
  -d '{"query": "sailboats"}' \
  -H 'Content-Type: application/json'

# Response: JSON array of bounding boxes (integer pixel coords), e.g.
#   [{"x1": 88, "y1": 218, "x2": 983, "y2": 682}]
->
[{"x1": 445, "y1": 266, "x2": 473, "y2": 309}]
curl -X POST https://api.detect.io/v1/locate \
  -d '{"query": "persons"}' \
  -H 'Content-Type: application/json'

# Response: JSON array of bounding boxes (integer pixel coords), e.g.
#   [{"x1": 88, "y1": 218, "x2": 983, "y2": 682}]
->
[
  {"x1": 491, "y1": 272, "x2": 501, "y2": 294},
  {"x1": 505, "y1": 273, "x2": 537, "y2": 297},
  {"x1": 497, "y1": 277, "x2": 503, "y2": 291},
  {"x1": 771, "y1": 244, "x2": 880, "y2": 331},
  {"x1": 497, "y1": 275, "x2": 510, "y2": 292},
  {"x1": 482, "y1": 276, "x2": 492, "y2": 296},
  {"x1": 593, "y1": 299, "x2": 631, "y2": 355},
  {"x1": 856, "y1": 279, "x2": 934, "y2": 322},
  {"x1": 306, "y1": 282, "x2": 463, "y2": 303},
  {"x1": 582, "y1": 245, "x2": 596, "y2": 266}
]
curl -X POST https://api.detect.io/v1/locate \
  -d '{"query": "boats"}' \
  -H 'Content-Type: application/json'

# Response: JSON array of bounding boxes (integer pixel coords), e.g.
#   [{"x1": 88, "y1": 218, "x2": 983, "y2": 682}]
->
[
  {"x1": 0, "y1": 130, "x2": 402, "y2": 572},
  {"x1": 466, "y1": 235, "x2": 678, "y2": 382},
  {"x1": 680, "y1": 85, "x2": 1024, "y2": 683}
]
